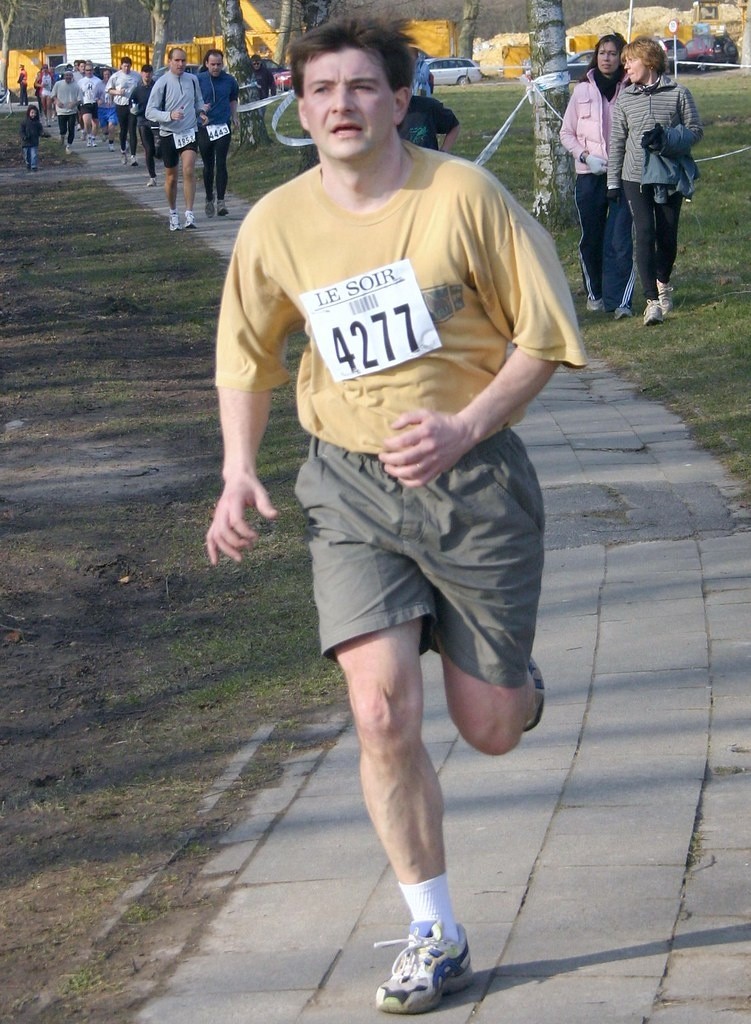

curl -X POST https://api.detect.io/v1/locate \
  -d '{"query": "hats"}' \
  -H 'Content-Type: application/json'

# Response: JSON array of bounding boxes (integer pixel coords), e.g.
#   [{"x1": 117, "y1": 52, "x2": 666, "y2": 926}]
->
[{"x1": 142, "y1": 65, "x2": 152, "y2": 72}]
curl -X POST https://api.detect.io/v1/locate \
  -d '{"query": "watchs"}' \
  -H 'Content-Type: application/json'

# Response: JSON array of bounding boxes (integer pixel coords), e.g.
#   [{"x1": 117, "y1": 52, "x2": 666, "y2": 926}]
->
[{"x1": 580, "y1": 157, "x2": 584, "y2": 163}]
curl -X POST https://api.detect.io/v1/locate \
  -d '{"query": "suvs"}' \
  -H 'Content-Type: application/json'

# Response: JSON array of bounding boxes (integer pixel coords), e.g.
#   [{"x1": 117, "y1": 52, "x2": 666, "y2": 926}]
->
[{"x1": 686, "y1": 34, "x2": 739, "y2": 72}]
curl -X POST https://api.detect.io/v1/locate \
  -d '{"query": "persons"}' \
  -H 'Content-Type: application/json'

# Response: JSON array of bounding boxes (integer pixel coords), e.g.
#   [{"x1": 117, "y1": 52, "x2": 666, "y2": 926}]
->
[
  {"x1": 17, "y1": 65, "x2": 28, "y2": 106},
  {"x1": 105, "y1": 57, "x2": 144, "y2": 166},
  {"x1": 146, "y1": 48, "x2": 208, "y2": 231},
  {"x1": 559, "y1": 32, "x2": 636, "y2": 319},
  {"x1": 129, "y1": 65, "x2": 162, "y2": 186},
  {"x1": 606, "y1": 38, "x2": 703, "y2": 324},
  {"x1": 50, "y1": 70, "x2": 82, "y2": 154},
  {"x1": 397, "y1": 95, "x2": 460, "y2": 153},
  {"x1": 34, "y1": 60, "x2": 101, "y2": 147},
  {"x1": 194, "y1": 49, "x2": 239, "y2": 218},
  {"x1": 18, "y1": 104, "x2": 51, "y2": 171},
  {"x1": 250, "y1": 55, "x2": 276, "y2": 115},
  {"x1": 205, "y1": 17, "x2": 589, "y2": 1013},
  {"x1": 411, "y1": 47, "x2": 431, "y2": 97},
  {"x1": 93, "y1": 69, "x2": 118, "y2": 152}
]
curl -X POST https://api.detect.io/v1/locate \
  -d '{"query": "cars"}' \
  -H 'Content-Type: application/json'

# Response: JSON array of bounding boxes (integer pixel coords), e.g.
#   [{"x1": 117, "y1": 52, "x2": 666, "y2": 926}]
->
[
  {"x1": 262, "y1": 58, "x2": 291, "y2": 76},
  {"x1": 568, "y1": 51, "x2": 597, "y2": 82},
  {"x1": 653, "y1": 37, "x2": 688, "y2": 74},
  {"x1": 424, "y1": 58, "x2": 482, "y2": 84},
  {"x1": 152, "y1": 64, "x2": 203, "y2": 82},
  {"x1": 273, "y1": 65, "x2": 293, "y2": 91}
]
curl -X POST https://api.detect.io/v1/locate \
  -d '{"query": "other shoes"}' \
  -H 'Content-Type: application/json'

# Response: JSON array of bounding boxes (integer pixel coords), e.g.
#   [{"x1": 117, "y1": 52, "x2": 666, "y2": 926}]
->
[
  {"x1": 26, "y1": 165, "x2": 31, "y2": 170},
  {"x1": 32, "y1": 167, "x2": 37, "y2": 172}
]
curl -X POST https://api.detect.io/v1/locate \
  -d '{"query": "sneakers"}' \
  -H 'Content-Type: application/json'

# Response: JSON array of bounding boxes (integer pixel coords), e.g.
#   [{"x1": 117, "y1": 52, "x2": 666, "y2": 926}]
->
[
  {"x1": 44, "y1": 118, "x2": 128, "y2": 166},
  {"x1": 217, "y1": 200, "x2": 229, "y2": 217},
  {"x1": 643, "y1": 300, "x2": 663, "y2": 325},
  {"x1": 184, "y1": 213, "x2": 197, "y2": 229},
  {"x1": 614, "y1": 307, "x2": 632, "y2": 321},
  {"x1": 587, "y1": 296, "x2": 604, "y2": 313},
  {"x1": 523, "y1": 656, "x2": 545, "y2": 729},
  {"x1": 656, "y1": 279, "x2": 674, "y2": 315},
  {"x1": 374, "y1": 919, "x2": 471, "y2": 1015},
  {"x1": 205, "y1": 194, "x2": 215, "y2": 218},
  {"x1": 146, "y1": 178, "x2": 156, "y2": 188},
  {"x1": 130, "y1": 156, "x2": 138, "y2": 166},
  {"x1": 169, "y1": 209, "x2": 182, "y2": 231}
]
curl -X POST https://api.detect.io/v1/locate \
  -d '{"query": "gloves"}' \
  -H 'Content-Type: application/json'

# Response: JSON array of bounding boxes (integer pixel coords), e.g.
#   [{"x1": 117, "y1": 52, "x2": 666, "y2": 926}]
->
[
  {"x1": 641, "y1": 122, "x2": 664, "y2": 152},
  {"x1": 586, "y1": 154, "x2": 607, "y2": 177},
  {"x1": 606, "y1": 189, "x2": 622, "y2": 209}
]
